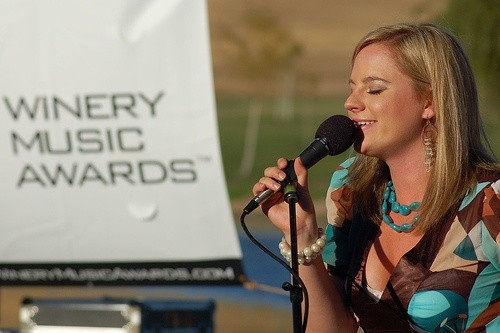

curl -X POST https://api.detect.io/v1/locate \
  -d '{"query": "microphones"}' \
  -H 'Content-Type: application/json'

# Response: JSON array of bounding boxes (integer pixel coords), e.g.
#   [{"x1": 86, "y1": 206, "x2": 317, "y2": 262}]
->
[{"x1": 242, "y1": 115, "x2": 374, "y2": 215}]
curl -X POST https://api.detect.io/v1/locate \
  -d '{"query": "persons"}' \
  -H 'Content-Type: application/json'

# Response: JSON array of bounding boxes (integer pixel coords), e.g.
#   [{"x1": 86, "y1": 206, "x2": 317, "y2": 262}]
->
[{"x1": 253, "y1": 23, "x2": 500, "y2": 333}]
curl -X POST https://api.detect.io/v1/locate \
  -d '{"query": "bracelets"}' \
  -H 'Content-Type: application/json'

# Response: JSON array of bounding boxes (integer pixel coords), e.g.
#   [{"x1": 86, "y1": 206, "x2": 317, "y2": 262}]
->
[{"x1": 278, "y1": 227, "x2": 327, "y2": 263}]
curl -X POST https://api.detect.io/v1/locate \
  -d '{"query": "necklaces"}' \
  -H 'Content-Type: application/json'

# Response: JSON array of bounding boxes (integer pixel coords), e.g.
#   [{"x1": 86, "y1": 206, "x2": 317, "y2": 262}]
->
[{"x1": 381, "y1": 181, "x2": 421, "y2": 233}]
